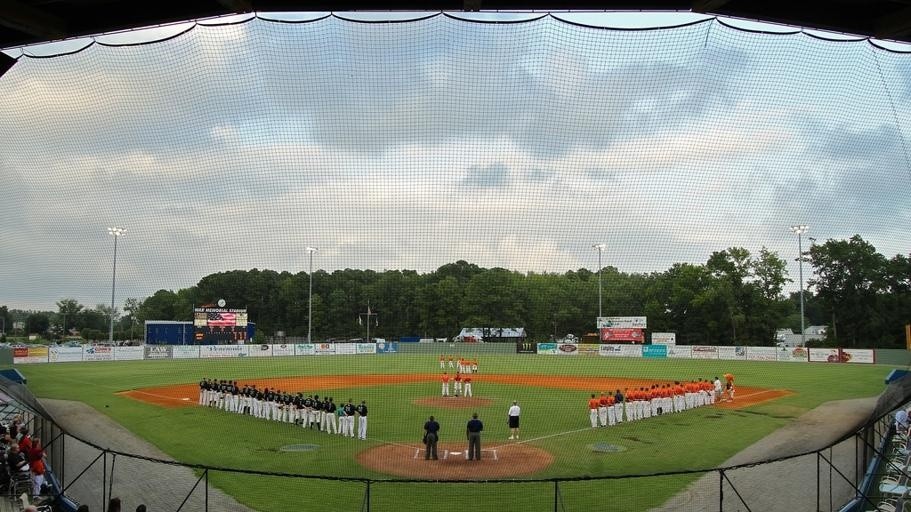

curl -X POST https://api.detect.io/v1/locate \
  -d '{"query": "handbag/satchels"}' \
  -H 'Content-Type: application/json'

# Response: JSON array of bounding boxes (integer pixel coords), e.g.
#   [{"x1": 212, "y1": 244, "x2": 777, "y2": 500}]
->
[{"x1": 423, "y1": 431, "x2": 428, "y2": 444}]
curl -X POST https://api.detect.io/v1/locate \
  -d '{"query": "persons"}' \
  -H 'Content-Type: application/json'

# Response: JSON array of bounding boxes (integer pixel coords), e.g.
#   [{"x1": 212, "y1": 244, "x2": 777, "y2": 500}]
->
[
  {"x1": 506, "y1": 398, "x2": 524, "y2": 440},
  {"x1": 422, "y1": 416, "x2": 440, "y2": 460},
  {"x1": 0, "y1": 411, "x2": 50, "y2": 511},
  {"x1": 435, "y1": 352, "x2": 485, "y2": 399},
  {"x1": 587, "y1": 368, "x2": 737, "y2": 430},
  {"x1": 466, "y1": 412, "x2": 483, "y2": 461},
  {"x1": 194, "y1": 374, "x2": 369, "y2": 442},
  {"x1": 76, "y1": 501, "x2": 90, "y2": 510},
  {"x1": 136, "y1": 503, "x2": 146, "y2": 510},
  {"x1": 106, "y1": 495, "x2": 121, "y2": 511}
]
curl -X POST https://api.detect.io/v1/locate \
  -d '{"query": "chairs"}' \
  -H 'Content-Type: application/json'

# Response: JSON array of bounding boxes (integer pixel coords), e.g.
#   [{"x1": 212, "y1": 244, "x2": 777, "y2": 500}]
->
[{"x1": 873, "y1": 411, "x2": 911, "y2": 512}]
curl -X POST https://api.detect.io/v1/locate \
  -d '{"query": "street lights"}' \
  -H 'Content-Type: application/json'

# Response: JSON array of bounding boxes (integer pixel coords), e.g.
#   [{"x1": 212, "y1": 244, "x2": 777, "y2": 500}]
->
[
  {"x1": 307, "y1": 245, "x2": 314, "y2": 341},
  {"x1": 592, "y1": 243, "x2": 604, "y2": 342},
  {"x1": 790, "y1": 226, "x2": 810, "y2": 346},
  {"x1": 109, "y1": 226, "x2": 125, "y2": 349}
]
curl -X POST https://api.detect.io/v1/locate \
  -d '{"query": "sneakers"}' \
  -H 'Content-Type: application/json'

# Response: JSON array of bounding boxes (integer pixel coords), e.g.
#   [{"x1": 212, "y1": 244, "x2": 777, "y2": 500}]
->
[
  {"x1": 425, "y1": 457, "x2": 438, "y2": 460},
  {"x1": 508, "y1": 435, "x2": 519, "y2": 440},
  {"x1": 592, "y1": 403, "x2": 714, "y2": 429},
  {"x1": 199, "y1": 403, "x2": 367, "y2": 441}
]
